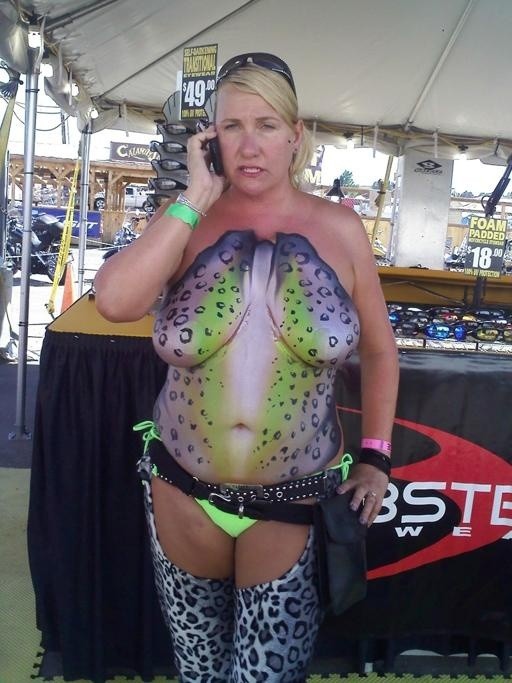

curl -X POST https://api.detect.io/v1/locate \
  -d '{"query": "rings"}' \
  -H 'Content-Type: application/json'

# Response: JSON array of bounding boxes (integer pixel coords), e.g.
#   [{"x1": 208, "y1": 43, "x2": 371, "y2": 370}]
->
[{"x1": 367, "y1": 492, "x2": 376, "y2": 498}]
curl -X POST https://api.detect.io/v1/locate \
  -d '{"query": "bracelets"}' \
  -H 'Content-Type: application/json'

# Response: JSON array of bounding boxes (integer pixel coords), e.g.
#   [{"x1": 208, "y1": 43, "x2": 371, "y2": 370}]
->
[
  {"x1": 358, "y1": 435, "x2": 393, "y2": 453},
  {"x1": 164, "y1": 202, "x2": 201, "y2": 228},
  {"x1": 356, "y1": 447, "x2": 391, "y2": 477},
  {"x1": 174, "y1": 191, "x2": 206, "y2": 218}
]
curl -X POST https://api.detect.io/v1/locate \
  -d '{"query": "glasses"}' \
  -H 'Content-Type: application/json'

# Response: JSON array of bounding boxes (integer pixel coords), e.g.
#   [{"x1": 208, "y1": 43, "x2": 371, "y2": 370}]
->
[
  {"x1": 387, "y1": 302, "x2": 512, "y2": 344},
  {"x1": 213, "y1": 53, "x2": 297, "y2": 97}
]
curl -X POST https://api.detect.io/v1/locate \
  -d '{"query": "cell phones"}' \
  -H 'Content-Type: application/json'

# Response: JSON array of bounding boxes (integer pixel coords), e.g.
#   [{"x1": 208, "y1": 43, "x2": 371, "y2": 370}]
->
[{"x1": 208, "y1": 121, "x2": 224, "y2": 176}]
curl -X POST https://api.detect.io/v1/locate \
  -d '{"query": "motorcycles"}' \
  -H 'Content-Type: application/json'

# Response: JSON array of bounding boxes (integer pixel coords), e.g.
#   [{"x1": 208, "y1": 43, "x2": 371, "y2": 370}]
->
[
  {"x1": 364, "y1": 228, "x2": 389, "y2": 259},
  {"x1": 3, "y1": 193, "x2": 73, "y2": 286},
  {"x1": 445, "y1": 235, "x2": 511, "y2": 275},
  {"x1": 100, "y1": 210, "x2": 150, "y2": 263}
]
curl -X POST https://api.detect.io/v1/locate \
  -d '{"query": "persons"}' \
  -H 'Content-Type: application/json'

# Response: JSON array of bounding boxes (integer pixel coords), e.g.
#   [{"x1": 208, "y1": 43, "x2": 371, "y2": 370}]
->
[{"x1": 93, "y1": 50, "x2": 401, "y2": 679}]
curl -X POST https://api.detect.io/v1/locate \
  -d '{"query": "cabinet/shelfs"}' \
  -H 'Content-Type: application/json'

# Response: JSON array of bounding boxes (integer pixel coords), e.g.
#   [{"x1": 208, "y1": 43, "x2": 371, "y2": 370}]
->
[{"x1": 30, "y1": 266, "x2": 512, "y2": 681}]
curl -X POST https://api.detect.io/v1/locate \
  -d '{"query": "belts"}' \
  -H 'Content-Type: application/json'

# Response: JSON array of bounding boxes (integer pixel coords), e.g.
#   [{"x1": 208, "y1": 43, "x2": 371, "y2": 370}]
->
[{"x1": 154, "y1": 466, "x2": 322, "y2": 505}]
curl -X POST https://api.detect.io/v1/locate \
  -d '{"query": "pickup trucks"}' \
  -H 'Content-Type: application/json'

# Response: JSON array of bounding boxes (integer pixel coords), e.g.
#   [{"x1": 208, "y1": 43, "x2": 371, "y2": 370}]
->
[{"x1": 93, "y1": 186, "x2": 156, "y2": 213}]
